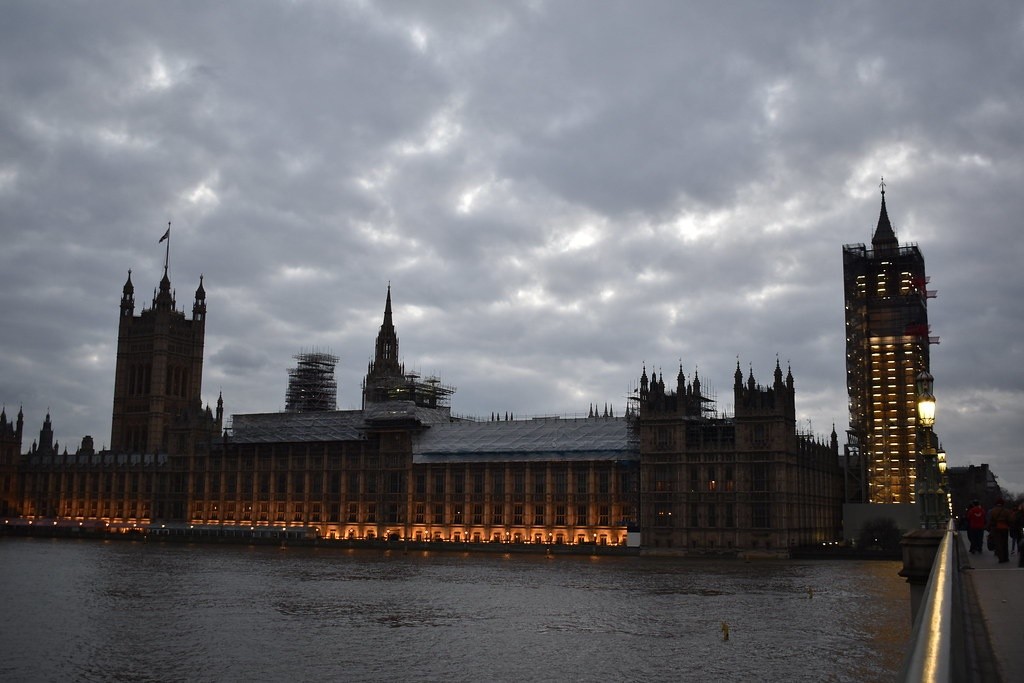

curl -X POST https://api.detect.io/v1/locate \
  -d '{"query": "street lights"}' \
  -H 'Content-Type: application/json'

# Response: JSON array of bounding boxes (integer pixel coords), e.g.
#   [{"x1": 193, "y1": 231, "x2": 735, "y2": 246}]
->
[
  {"x1": 349, "y1": 528, "x2": 354, "y2": 539},
  {"x1": 387, "y1": 528, "x2": 390, "y2": 541},
  {"x1": 251, "y1": 525, "x2": 254, "y2": 539},
  {"x1": 190, "y1": 525, "x2": 194, "y2": 537},
  {"x1": 79, "y1": 520, "x2": 83, "y2": 532},
  {"x1": 161, "y1": 523, "x2": 165, "y2": 536},
  {"x1": 106, "y1": 521, "x2": 109, "y2": 535},
  {"x1": 316, "y1": 528, "x2": 319, "y2": 540},
  {"x1": 133, "y1": 524, "x2": 136, "y2": 535},
  {"x1": 918, "y1": 359, "x2": 942, "y2": 527},
  {"x1": 425, "y1": 528, "x2": 429, "y2": 542},
  {"x1": 938, "y1": 444, "x2": 946, "y2": 523},
  {"x1": 465, "y1": 530, "x2": 468, "y2": 543},
  {"x1": 549, "y1": 531, "x2": 552, "y2": 545},
  {"x1": 282, "y1": 526, "x2": 286, "y2": 539},
  {"x1": 947, "y1": 491, "x2": 953, "y2": 518},
  {"x1": 28, "y1": 519, "x2": 32, "y2": 531},
  {"x1": 506, "y1": 531, "x2": 510, "y2": 543},
  {"x1": 593, "y1": 533, "x2": 597, "y2": 546},
  {"x1": 5, "y1": 519, "x2": 9, "y2": 529},
  {"x1": 54, "y1": 519, "x2": 58, "y2": 530}
]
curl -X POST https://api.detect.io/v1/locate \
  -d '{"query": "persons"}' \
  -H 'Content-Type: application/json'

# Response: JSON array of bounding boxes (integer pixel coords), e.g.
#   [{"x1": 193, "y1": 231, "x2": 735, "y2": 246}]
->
[
  {"x1": 959, "y1": 499, "x2": 989, "y2": 554},
  {"x1": 1010, "y1": 499, "x2": 1024, "y2": 568},
  {"x1": 988, "y1": 498, "x2": 1016, "y2": 563}
]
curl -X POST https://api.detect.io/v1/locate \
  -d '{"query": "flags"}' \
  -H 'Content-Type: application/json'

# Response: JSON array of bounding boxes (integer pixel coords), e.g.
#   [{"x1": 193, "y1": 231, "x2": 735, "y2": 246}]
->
[{"x1": 159, "y1": 229, "x2": 169, "y2": 243}]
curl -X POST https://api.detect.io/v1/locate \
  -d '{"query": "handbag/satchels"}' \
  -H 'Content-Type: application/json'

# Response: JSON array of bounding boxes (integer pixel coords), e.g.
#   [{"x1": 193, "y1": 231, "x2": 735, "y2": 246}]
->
[{"x1": 987, "y1": 535, "x2": 994, "y2": 551}]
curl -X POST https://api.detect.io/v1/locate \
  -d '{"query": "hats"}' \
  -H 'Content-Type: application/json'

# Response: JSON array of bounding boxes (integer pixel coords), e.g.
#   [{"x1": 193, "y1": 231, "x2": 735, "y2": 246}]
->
[{"x1": 994, "y1": 499, "x2": 1004, "y2": 506}]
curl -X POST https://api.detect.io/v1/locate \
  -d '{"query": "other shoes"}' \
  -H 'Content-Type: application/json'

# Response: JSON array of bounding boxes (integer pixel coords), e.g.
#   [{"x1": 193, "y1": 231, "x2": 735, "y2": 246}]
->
[
  {"x1": 999, "y1": 559, "x2": 1009, "y2": 562},
  {"x1": 972, "y1": 549, "x2": 975, "y2": 554},
  {"x1": 1010, "y1": 551, "x2": 1015, "y2": 555}
]
